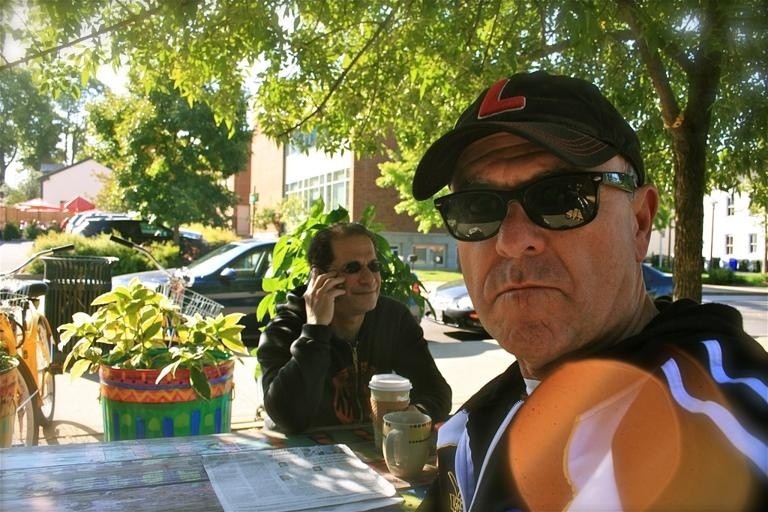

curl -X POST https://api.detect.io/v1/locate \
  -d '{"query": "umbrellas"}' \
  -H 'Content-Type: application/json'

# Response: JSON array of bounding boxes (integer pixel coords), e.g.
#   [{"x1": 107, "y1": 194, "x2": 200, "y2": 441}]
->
[
  {"x1": 65, "y1": 196, "x2": 96, "y2": 213},
  {"x1": 16, "y1": 198, "x2": 63, "y2": 221}
]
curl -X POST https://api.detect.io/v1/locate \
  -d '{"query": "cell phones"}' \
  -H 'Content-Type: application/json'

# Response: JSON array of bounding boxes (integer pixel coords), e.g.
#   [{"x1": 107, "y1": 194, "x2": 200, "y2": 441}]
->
[{"x1": 306, "y1": 265, "x2": 342, "y2": 302}]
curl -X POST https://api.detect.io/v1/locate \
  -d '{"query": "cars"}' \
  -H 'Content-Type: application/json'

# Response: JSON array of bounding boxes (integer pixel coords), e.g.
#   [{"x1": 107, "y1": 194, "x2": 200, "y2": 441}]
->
[
  {"x1": 19, "y1": 236, "x2": 423, "y2": 349},
  {"x1": 425, "y1": 262, "x2": 682, "y2": 343}
]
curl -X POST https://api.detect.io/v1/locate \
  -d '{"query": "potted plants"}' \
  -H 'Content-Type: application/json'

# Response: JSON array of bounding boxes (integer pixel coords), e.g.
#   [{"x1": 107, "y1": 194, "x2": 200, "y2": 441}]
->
[{"x1": 58, "y1": 276, "x2": 248, "y2": 443}]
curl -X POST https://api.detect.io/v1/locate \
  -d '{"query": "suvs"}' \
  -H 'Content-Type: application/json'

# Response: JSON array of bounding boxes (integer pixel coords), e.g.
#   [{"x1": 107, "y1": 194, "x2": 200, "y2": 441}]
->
[{"x1": 60, "y1": 207, "x2": 211, "y2": 256}]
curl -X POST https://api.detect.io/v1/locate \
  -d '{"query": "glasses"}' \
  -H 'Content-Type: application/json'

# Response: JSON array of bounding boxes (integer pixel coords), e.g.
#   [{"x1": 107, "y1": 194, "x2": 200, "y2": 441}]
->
[
  {"x1": 341, "y1": 259, "x2": 382, "y2": 274},
  {"x1": 434, "y1": 171, "x2": 635, "y2": 242}
]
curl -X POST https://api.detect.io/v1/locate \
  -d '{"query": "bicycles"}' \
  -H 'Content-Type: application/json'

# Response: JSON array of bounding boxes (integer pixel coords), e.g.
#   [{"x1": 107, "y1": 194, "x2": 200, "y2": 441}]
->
[
  {"x1": 1, "y1": 281, "x2": 56, "y2": 447},
  {"x1": 109, "y1": 236, "x2": 226, "y2": 346}
]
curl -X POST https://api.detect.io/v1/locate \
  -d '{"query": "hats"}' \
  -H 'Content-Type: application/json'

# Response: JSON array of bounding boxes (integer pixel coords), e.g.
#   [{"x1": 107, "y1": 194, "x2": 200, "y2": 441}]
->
[{"x1": 412, "y1": 70, "x2": 644, "y2": 201}]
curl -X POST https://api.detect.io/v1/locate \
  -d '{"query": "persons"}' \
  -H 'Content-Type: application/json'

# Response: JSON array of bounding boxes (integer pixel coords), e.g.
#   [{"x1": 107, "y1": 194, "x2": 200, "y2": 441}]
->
[
  {"x1": 257, "y1": 222, "x2": 453, "y2": 433},
  {"x1": 414, "y1": 70, "x2": 767, "y2": 512},
  {"x1": 19, "y1": 217, "x2": 70, "y2": 231}
]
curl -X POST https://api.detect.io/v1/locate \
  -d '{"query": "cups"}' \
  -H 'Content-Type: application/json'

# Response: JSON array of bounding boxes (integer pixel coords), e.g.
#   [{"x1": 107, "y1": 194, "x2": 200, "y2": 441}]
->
[
  {"x1": 368, "y1": 374, "x2": 414, "y2": 452},
  {"x1": 381, "y1": 410, "x2": 433, "y2": 479}
]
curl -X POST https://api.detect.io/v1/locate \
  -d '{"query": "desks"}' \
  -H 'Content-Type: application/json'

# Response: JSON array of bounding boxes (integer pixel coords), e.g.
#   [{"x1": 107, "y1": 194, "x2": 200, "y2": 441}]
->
[{"x1": 2, "y1": 413, "x2": 449, "y2": 512}]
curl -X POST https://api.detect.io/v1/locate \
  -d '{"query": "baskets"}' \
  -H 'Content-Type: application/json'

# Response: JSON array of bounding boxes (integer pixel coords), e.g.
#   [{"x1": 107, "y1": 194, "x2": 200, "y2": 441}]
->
[
  {"x1": 160, "y1": 283, "x2": 225, "y2": 338},
  {"x1": 0, "y1": 278, "x2": 28, "y2": 314}
]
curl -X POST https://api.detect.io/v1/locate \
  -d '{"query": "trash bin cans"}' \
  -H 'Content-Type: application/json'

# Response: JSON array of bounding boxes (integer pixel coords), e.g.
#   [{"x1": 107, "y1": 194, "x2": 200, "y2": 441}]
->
[{"x1": 41, "y1": 256, "x2": 120, "y2": 373}]
